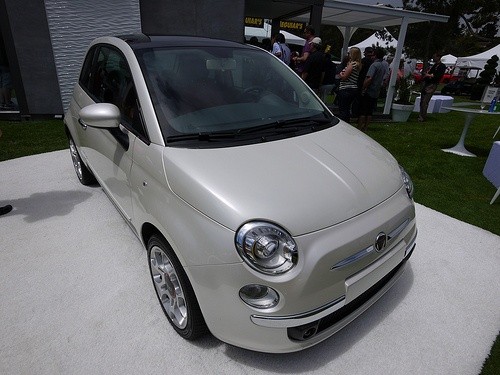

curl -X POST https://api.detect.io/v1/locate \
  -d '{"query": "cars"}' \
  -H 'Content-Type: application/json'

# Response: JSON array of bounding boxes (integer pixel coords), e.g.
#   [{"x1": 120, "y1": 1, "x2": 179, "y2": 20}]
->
[{"x1": 62, "y1": 35, "x2": 418, "y2": 354}]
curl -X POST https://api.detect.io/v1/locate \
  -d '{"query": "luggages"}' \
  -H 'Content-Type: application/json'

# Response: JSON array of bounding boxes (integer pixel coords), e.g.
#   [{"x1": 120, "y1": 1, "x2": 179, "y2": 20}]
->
[{"x1": 294, "y1": 59, "x2": 302, "y2": 77}]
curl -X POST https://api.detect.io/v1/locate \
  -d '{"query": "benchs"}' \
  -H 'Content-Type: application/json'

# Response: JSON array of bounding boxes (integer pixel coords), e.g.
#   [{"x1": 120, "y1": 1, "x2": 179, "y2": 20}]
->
[{"x1": 415, "y1": 95, "x2": 454, "y2": 114}]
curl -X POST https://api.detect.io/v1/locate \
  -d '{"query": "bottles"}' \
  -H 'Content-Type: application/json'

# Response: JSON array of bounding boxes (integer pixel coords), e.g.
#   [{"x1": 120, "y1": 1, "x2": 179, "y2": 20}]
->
[{"x1": 488, "y1": 96, "x2": 496, "y2": 112}]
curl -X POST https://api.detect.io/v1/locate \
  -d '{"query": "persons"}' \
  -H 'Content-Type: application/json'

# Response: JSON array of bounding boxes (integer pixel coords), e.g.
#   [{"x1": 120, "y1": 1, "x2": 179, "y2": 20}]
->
[{"x1": 244, "y1": 25, "x2": 454, "y2": 135}]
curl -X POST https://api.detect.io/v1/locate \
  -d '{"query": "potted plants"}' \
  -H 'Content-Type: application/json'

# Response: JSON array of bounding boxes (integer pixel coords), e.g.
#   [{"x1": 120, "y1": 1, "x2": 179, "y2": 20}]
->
[{"x1": 392, "y1": 72, "x2": 415, "y2": 123}]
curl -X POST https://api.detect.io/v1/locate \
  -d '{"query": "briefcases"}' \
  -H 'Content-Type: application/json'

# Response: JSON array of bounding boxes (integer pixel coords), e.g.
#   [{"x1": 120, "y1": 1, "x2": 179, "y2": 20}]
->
[{"x1": 357, "y1": 85, "x2": 377, "y2": 112}]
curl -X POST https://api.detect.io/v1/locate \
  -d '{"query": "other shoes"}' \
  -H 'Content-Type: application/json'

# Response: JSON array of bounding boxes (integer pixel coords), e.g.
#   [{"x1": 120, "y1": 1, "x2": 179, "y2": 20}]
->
[{"x1": 417, "y1": 115, "x2": 427, "y2": 121}]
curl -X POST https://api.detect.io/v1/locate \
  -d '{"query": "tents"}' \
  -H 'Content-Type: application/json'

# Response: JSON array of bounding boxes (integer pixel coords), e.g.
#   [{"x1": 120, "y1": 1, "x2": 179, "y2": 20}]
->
[
  {"x1": 452, "y1": 43, "x2": 500, "y2": 78},
  {"x1": 341, "y1": 26, "x2": 399, "y2": 70}
]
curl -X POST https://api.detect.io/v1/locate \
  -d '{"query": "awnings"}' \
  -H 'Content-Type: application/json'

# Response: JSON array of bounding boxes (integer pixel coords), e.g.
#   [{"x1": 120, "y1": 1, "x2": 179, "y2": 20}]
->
[{"x1": 264, "y1": 0, "x2": 452, "y2": 118}]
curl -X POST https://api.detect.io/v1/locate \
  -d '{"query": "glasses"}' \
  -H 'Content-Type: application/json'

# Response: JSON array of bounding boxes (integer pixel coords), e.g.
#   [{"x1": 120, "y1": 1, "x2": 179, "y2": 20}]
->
[
  {"x1": 365, "y1": 50, "x2": 374, "y2": 54},
  {"x1": 432, "y1": 56, "x2": 439, "y2": 58},
  {"x1": 371, "y1": 54, "x2": 376, "y2": 57}
]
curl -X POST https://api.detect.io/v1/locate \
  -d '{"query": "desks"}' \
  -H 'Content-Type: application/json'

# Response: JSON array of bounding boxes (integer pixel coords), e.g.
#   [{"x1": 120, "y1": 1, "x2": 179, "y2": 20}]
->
[{"x1": 440, "y1": 106, "x2": 500, "y2": 157}]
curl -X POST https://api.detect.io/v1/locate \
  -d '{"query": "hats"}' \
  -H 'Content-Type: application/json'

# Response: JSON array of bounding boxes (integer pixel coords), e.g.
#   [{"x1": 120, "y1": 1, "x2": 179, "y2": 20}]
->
[{"x1": 308, "y1": 36, "x2": 323, "y2": 45}]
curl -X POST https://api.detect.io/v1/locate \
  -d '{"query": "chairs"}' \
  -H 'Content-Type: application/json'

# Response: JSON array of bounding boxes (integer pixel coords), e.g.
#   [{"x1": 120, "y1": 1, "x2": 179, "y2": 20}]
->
[{"x1": 174, "y1": 59, "x2": 223, "y2": 115}]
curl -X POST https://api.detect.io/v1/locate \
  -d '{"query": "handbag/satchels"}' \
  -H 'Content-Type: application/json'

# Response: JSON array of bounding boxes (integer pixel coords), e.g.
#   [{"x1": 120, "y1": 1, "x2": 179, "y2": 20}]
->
[
  {"x1": 337, "y1": 89, "x2": 358, "y2": 101},
  {"x1": 278, "y1": 42, "x2": 287, "y2": 63}
]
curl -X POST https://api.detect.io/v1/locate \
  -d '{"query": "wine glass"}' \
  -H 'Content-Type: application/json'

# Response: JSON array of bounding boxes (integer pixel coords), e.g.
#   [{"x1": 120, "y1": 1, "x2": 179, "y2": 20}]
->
[{"x1": 480, "y1": 103, "x2": 486, "y2": 112}]
